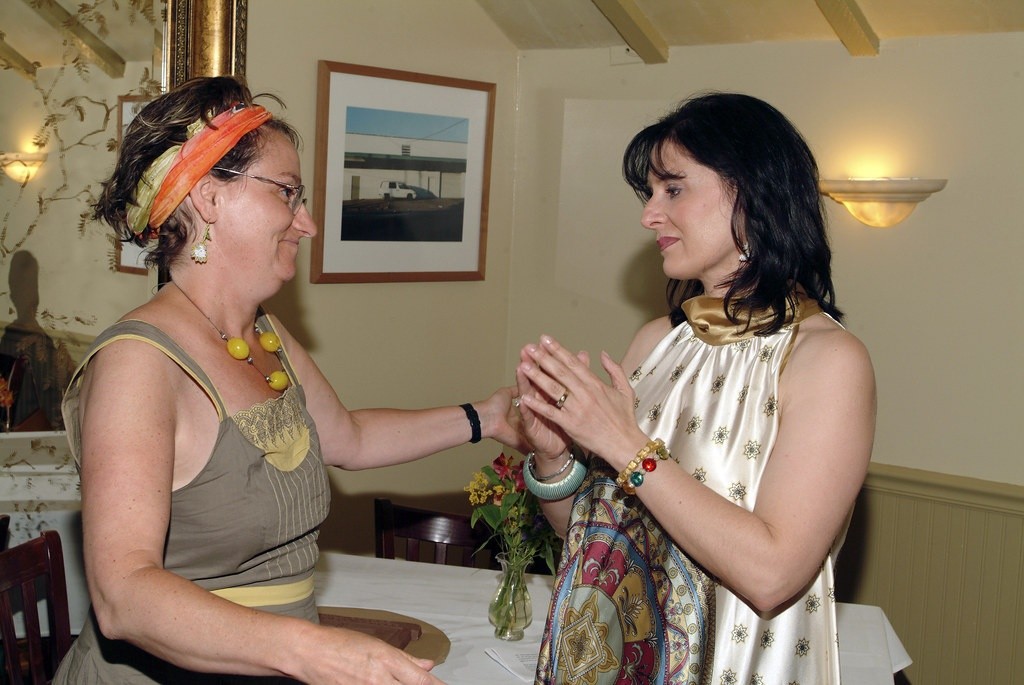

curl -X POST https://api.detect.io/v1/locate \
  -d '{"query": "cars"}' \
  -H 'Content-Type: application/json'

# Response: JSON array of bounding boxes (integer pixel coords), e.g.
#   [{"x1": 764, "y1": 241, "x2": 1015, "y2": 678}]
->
[{"x1": 379, "y1": 180, "x2": 425, "y2": 201}]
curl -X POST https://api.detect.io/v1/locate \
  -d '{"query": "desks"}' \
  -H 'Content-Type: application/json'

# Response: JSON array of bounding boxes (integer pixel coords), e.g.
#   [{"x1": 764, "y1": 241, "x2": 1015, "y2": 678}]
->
[{"x1": 315, "y1": 552, "x2": 914, "y2": 685}]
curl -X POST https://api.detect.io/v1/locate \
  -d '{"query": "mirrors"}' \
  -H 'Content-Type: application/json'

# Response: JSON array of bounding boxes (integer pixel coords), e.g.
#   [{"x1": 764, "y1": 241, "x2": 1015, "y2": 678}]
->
[{"x1": 0, "y1": 0, "x2": 249, "y2": 519}]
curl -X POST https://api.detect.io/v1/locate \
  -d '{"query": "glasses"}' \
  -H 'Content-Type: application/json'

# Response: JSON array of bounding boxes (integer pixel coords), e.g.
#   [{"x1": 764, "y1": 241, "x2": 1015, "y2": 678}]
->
[{"x1": 212, "y1": 167, "x2": 308, "y2": 216}]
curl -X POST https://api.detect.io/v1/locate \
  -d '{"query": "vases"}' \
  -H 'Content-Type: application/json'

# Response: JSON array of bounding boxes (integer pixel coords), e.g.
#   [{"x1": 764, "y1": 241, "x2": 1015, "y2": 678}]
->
[{"x1": 488, "y1": 551, "x2": 535, "y2": 642}]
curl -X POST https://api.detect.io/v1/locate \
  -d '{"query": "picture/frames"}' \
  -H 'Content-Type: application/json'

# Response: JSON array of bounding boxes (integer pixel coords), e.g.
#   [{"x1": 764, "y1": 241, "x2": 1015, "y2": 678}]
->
[
  {"x1": 115, "y1": 95, "x2": 160, "y2": 276},
  {"x1": 309, "y1": 60, "x2": 497, "y2": 283}
]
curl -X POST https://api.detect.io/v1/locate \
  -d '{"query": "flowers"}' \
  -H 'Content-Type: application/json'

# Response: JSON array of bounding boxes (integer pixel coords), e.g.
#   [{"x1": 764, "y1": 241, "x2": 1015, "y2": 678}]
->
[{"x1": 462, "y1": 453, "x2": 562, "y2": 576}]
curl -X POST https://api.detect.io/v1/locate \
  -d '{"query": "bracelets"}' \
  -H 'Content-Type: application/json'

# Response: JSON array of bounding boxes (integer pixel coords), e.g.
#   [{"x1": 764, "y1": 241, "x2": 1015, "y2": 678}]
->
[
  {"x1": 459, "y1": 403, "x2": 481, "y2": 443},
  {"x1": 523, "y1": 444, "x2": 588, "y2": 500},
  {"x1": 615, "y1": 437, "x2": 671, "y2": 495}
]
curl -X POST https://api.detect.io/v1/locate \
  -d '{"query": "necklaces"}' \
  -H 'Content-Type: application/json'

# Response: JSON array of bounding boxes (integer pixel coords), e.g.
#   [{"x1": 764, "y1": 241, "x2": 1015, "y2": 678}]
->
[{"x1": 171, "y1": 279, "x2": 289, "y2": 391}]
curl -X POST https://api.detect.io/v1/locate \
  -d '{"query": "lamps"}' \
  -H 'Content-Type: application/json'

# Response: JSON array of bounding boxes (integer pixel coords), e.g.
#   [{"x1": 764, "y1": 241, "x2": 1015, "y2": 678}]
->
[
  {"x1": 0, "y1": 150, "x2": 48, "y2": 183},
  {"x1": 817, "y1": 175, "x2": 948, "y2": 228}
]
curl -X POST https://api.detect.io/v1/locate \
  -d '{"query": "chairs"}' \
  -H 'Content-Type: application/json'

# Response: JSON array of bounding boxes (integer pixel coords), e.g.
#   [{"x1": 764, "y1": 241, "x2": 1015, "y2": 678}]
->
[
  {"x1": 374, "y1": 498, "x2": 503, "y2": 571},
  {"x1": 0, "y1": 530, "x2": 71, "y2": 685}
]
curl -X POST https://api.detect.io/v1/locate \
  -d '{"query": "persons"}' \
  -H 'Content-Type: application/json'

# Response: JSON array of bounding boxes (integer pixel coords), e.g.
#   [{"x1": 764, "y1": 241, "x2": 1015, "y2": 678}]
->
[
  {"x1": 51, "y1": 76, "x2": 534, "y2": 684},
  {"x1": 514, "y1": 92, "x2": 877, "y2": 684}
]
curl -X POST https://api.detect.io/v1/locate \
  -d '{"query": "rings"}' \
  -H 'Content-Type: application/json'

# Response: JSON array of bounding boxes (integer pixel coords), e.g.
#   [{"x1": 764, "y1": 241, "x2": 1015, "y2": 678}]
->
[
  {"x1": 556, "y1": 388, "x2": 570, "y2": 409},
  {"x1": 512, "y1": 394, "x2": 521, "y2": 409}
]
